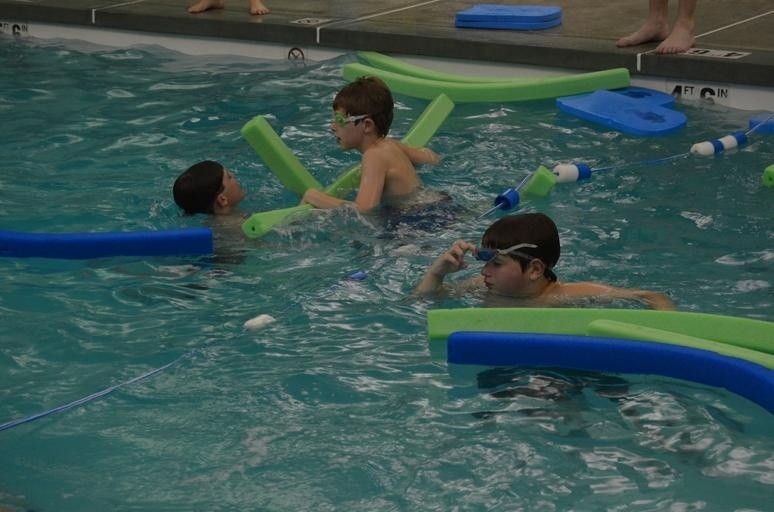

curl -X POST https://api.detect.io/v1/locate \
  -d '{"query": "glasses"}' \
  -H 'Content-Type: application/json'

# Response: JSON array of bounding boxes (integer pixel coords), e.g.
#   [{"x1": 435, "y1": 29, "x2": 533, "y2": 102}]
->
[
  {"x1": 473, "y1": 242, "x2": 538, "y2": 263},
  {"x1": 334, "y1": 111, "x2": 370, "y2": 127}
]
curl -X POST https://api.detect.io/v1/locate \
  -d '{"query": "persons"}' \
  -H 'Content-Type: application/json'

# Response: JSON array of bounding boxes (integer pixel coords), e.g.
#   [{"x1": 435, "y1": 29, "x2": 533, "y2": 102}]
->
[
  {"x1": 615, "y1": 0, "x2": 699, "y2": 55},
  {"x1": 412, "y1": 213, "x2": 676, "y2": 311},
  {"x1": 173, "y1": 160, "x2": 246, "y2": 265},
  {"x1": 300, "y1": 76, "x2": 474, "y2": 241},
  {"x1": 188, "y1": 0, "x2": 270, "y2": 15}
]
